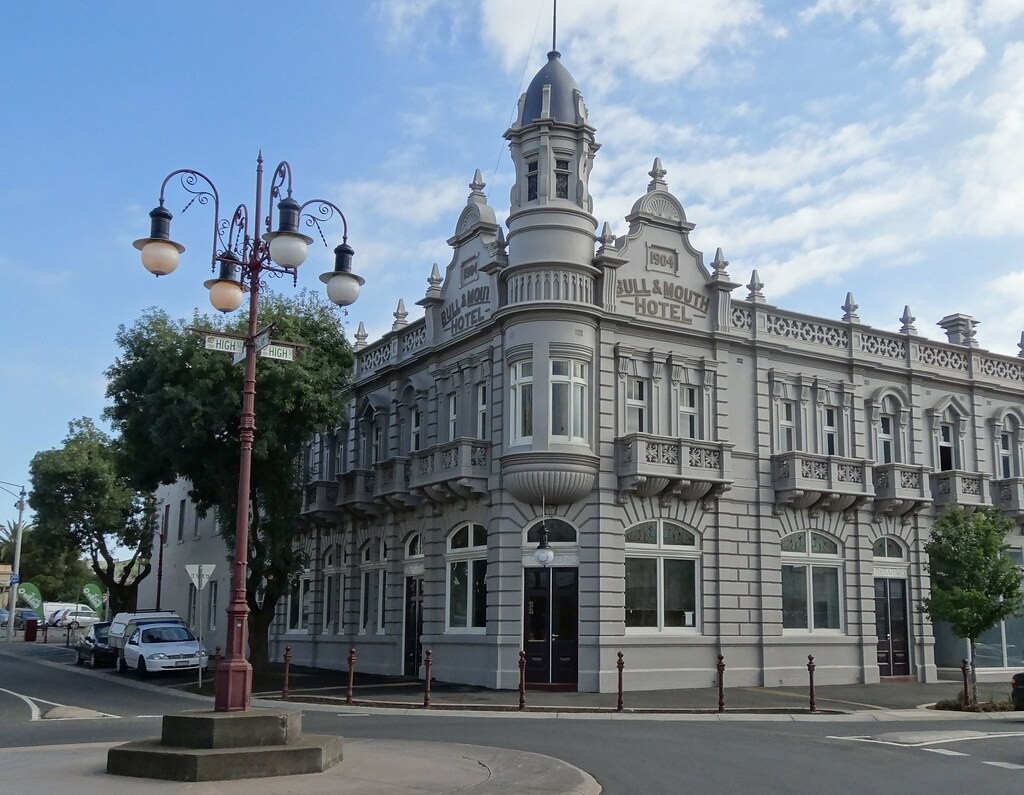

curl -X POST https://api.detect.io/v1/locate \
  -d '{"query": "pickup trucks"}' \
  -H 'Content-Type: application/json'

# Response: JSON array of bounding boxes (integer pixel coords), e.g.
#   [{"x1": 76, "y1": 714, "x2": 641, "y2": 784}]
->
[{"x1": 106, "y1": 607, "x2": 209, "y2": 680}]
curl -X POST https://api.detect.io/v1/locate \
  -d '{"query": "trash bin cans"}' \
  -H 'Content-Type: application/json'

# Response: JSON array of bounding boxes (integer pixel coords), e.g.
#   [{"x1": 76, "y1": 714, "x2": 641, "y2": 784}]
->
[{"x1": 25, "y1": 618, "x2": 38, "y2": 641}]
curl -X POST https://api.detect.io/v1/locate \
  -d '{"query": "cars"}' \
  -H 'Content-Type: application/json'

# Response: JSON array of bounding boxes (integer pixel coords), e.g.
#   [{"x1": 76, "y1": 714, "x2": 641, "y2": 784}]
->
[
  {"x1": 14, "y1": 600, "x2": 101, "y2": 630},
  {"x1": 0, "y1": 607, "x2": 10, "y2": 627},
  {"x1": 974, "y1": 640, "x2": 1024, "y2": 668},
  {"x1": 74, "y1": 621, "x2": 119, "y2": 669}
]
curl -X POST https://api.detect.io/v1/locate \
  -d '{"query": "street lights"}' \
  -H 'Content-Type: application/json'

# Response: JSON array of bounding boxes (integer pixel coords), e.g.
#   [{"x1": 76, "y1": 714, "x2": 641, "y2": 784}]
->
[
  {"x1": 131, "y1": 148, "x2": 366, "y2": 714},
  {"x1": 135, "y1": 513, "x2": 166, "y2": 612}
]
